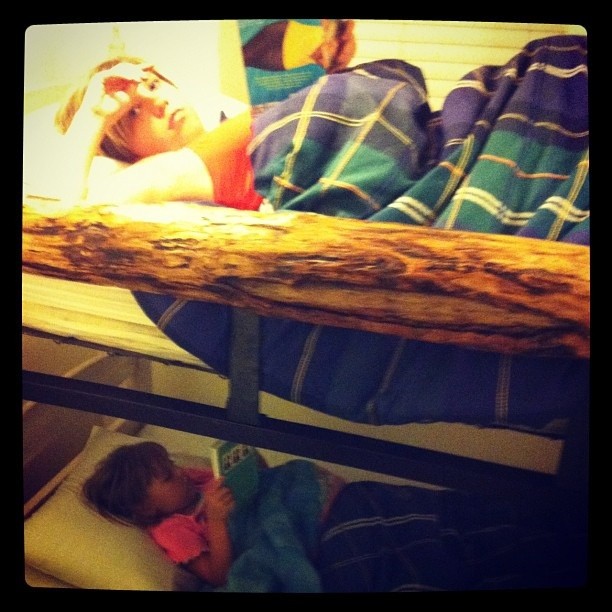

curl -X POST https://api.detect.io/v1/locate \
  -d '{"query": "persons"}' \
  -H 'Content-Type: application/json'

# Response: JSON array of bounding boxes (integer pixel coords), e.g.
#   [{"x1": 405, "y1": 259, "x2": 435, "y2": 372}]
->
[
  {"x1": 54, "y1": 21, "x2": 360, "y2": 214},
  {"x1": 79, "y1": 440, "x2": 238, "y2": 588}
]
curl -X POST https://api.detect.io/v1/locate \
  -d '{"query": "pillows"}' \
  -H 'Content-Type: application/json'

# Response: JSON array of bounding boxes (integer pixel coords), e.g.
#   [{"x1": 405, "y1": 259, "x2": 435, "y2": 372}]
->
[
  {"x1": 22, "y1": 77, "x2": 250, "y2": 198},
  {"x1": 25, "y1": 422, "x2": 212, "y2": 591}
]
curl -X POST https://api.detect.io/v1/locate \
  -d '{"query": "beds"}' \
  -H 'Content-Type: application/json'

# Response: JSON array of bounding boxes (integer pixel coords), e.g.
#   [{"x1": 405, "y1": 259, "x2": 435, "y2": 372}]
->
[{"x1": 22, "y1": 21, "x2": 591, "y2": 592}]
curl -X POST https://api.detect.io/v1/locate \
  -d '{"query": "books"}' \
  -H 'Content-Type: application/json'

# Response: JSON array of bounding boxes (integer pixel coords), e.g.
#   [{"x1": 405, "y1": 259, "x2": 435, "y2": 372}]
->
[
  {"x1": 208, "y1": 437, "x2": 263, "y2": 512},
  {"x1": 233, "y1": 18, "x2": 330, "y2": 119}
]
